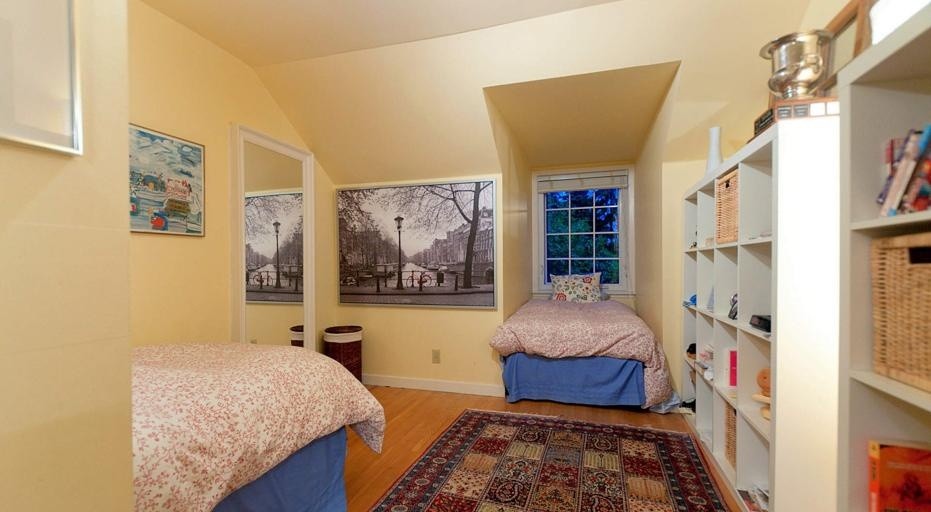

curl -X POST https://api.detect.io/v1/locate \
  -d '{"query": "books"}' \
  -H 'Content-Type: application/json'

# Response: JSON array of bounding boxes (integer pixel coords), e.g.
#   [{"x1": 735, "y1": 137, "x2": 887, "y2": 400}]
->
[
  {"x1": 875, "y1": 123, "x2": 931, "y2": 217},
  {"x1": 866, "y1": 435, "x2": 931, "y2": 512}
]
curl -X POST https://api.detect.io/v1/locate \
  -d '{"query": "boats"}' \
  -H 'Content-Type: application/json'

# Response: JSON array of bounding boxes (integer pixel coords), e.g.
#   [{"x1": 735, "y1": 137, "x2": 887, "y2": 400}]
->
[
  {"x1": 416, "y1": 261, "x2": 456, "y2": 274},
  {"x1": 274, "y1": 263, "x2": 301, "y2": 278},
  {"x1": 342, "y1": 262, "x2": 405, "y2": 286},
  {"x1": 246, "y1": 262, "x2": 267, "y2": 272}
]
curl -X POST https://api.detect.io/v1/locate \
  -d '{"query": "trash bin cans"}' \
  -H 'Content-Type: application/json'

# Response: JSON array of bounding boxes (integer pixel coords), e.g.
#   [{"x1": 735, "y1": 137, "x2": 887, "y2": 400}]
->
[
  {"x1": 323, "y1": 326, "x2": 363, "y2": 382},
  {"x1": 289, "y1": 325, "x2": 304, "y2": 346}
]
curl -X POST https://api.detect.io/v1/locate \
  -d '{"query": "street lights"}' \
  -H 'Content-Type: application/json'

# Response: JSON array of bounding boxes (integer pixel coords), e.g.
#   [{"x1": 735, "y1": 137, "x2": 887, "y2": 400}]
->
[
  {"x1": 395, "y1": 215, "x2": 404, "y2": 289},
  {"x1": 273, "y1": 221, "x2": 283, "y2": 287}
]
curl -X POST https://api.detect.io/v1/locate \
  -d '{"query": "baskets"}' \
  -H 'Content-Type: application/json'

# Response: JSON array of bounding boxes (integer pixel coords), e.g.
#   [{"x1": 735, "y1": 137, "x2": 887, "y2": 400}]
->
[
  {"x1": 871, "y1": 231, "x2": 931, "y2": 397},
  {"x1": 717, "y1": 167, "x2": 738, "y2": 244}
]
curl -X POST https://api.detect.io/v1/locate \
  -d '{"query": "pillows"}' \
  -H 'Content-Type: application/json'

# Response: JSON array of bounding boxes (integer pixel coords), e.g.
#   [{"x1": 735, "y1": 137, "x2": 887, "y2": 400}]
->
[{"x1": 550, "y1": 272, "x2": 603, "y2": 303}]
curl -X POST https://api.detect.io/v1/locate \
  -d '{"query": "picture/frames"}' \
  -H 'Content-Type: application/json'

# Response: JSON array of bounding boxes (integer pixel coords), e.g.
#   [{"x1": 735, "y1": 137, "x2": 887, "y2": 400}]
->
[
  {"x1": 2, "y1": 1, "x2": 84, "y2": 159},
  {"x1": 336, "y1": 177, "x2": 498, "y2": 310},
  {"x1": 129, "y1": 123, "x2": 207, "y2": 237},
  {"x1": 246, "y1": 191, "x2": 304, "y2": 305}
]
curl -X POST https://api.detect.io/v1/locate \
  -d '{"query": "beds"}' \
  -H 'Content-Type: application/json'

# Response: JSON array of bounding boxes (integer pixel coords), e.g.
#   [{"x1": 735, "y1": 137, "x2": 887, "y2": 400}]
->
[
  {"x1": 505, "y1": 296, "x2": 645, "y2": 413},
  {"x1": 132, "y1": 345, "x2": 350, "y2": 512}
]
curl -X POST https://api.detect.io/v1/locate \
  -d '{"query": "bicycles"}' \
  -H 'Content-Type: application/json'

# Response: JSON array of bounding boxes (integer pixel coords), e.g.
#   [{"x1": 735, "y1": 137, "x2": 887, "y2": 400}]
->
[
  {"x1": 253, "y1": 271, "x2": 273, "y2": 287},
  {"x1": 407, "y1": 270, "x2": 434, "y2": 288}
]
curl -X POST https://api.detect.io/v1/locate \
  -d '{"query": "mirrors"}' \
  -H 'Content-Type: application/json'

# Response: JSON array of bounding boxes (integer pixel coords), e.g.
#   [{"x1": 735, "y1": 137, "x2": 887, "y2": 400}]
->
[{"x1": 232, "y1": 123, "x2": 317, "y2": 352}]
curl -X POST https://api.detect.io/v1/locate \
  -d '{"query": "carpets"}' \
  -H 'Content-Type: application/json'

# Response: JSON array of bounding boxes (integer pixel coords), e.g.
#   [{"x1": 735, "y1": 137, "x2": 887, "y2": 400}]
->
[{"x1": 369, "y1": 406, "x2": 729, "y2": 510}]
